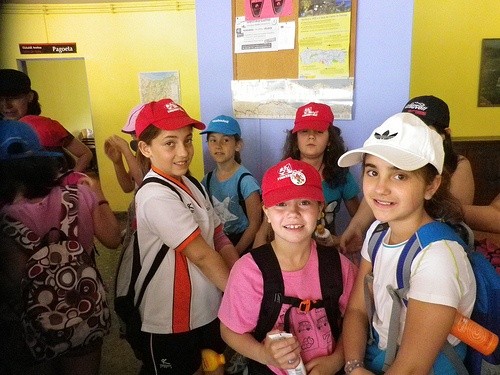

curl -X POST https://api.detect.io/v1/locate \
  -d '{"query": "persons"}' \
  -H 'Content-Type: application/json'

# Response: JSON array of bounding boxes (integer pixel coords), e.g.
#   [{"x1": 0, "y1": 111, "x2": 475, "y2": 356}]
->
[
  {"x1": 341, "y1": 95, "x2": 475, "y2": 259},
  {"x1": 0, "y1": 119, "x2": 121, "y2": 375},
  {"x1": 104, "y1": 106, "x2": 151, "y2": 192},
  {"x1": 133, "y1": 98, "x2": 239, "y2": 375},
  {"x1": 457, "y1": 193, "x2": 500, "y2": 275},
  {"x1": 217, "y1": 159, "x2": 360, "y2": 375},
  {"x1": 198, "y1": 115, "x2": 263, "y2": 254},
  {"x1": 338, "y1": 113, "x2": 476, "y2": 375},
  {"x1": 0, "y1": 69, "x2": 93, "y2": 173},
  {"x1": 251, "y1": 101, "x2": 361, "y2": 251}
]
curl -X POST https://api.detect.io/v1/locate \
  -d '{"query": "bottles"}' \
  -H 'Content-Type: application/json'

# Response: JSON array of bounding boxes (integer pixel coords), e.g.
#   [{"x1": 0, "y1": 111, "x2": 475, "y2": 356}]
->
[
  {"x1": 314, "y1": 224, "x2": 334, "y2": 246},
  {"x1": 200, "y1": 349, "x2": 226, "y2": 372},
  {"x1": 450, "y1": 312, "x2": 499, "y2": 356}
]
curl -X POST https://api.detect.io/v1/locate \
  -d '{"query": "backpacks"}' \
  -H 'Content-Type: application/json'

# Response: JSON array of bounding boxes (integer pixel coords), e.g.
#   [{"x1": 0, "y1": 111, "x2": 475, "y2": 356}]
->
[
  {"x1": 110, "y1": 178, "x2": 186, "y2": 338},
  {"x1": 0, "y1": 185, "x2": 112, "y2": 365},
  {"x1": 365, "y1": 221, "x2": 499, "y2": 375}
]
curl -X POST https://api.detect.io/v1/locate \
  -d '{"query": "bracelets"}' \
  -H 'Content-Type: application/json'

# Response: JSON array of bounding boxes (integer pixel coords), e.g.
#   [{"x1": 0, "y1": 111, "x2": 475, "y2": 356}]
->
[
  {"x1": 344, "y1": 360, "x2": 364, "y2": 375},
  {"x1": 98, "y1": 200, "x2": 109, "y2": 205}
]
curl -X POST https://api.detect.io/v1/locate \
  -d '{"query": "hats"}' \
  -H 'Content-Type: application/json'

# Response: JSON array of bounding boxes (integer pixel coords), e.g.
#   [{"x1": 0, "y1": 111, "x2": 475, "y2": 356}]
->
[
  {"x1": 291, "y1": 102, "x2": 335, "y2": 133},
  {"x1": 0, "y1": 68, "x2": 32, "y2": 95},
  {"x1": 402, "y1": 95, "x2": 451, "y2": 132},
  {"x1": 0, "y1": 118, "x2": 63, "y2": 158},
  {"x1": 259, "y1": 155, "x2": 326, "y2": 207},
  {"x1": 134, "y1": 98, "x2": 208, "y2": 133},
  {"x1": 338, "y1": 112, "x2": 445, "y2": 175},
  {"x1": 198, "y1": 114, "x2": 241, "y2": 138},
  {"x1": 120, "y1": 104, "x2": 149, "y2": 133}
]
